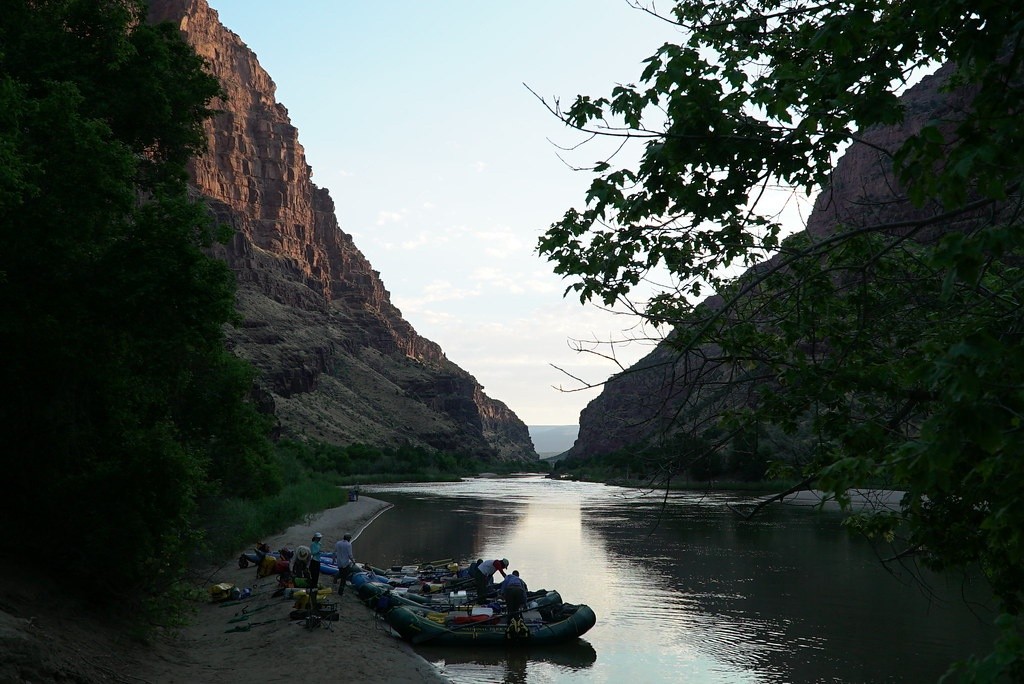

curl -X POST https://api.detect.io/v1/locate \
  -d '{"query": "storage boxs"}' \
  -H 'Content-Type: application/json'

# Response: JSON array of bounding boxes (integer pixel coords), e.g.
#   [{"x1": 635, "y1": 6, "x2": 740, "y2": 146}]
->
[
  {"x1": 318, "y1": 601, "x2": 338, "y2": 612},
  {"x1": 450, "y1": 590, "x2": 467, "y2": 601}
]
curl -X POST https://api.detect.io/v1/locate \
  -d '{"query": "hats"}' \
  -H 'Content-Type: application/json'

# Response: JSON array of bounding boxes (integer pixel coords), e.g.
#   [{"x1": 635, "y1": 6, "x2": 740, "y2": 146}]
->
[
  {"x1": 502, "y1": 559, "x2": 508, "y2": 569},
  {"x1": 314, "y1": 532, "x2": 323, "y2": 538},
  {"x1": 297, "y1": 549, "x2": 308, "y2": 561},
  {"x1": 344, "y1": 532, "x2": 352, "y2": 538}
]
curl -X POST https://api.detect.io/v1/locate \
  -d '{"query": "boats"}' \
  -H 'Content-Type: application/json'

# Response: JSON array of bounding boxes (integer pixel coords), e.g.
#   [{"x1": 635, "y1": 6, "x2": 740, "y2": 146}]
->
[{"x1": 244, "y1": 547, "x2": 597, "y2": 650}]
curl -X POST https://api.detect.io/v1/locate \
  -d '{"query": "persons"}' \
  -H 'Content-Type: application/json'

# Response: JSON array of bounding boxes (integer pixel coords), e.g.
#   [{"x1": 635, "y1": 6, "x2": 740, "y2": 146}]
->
[
  {"x1": 309, "y1": 532, "x2": 325, "y2": 588},
  {"x1": 331, "y1": 533, "x2": 355, "y2": 594},
  {"x1": 293, "y1": 545, "x2": 311, "y2": 578},
  {"x1": 354, "y1": 482, "x2": 359, "y2": 500},
  {"x1": 469, "y1": 557, "x2": 542, "y2": 631}
]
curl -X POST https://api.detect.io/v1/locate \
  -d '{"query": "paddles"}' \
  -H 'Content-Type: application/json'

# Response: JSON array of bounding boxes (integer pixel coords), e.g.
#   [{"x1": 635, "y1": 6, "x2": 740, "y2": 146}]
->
[
  {"x1": 416, "y1": 558, "x2": 456, "y2": 569},
  {"x1": 451, "y1": 602, "x2": 550, "y2": 631},
  {"x1": 426, "y1": 577, "x2": 476, "y2": 594}
]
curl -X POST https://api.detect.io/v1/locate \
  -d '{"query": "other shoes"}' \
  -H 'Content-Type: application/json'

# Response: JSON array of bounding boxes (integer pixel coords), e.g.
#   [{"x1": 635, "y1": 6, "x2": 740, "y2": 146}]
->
[{"x1": 337, "y1": 593, "x2": 345, "y2": 598}]
